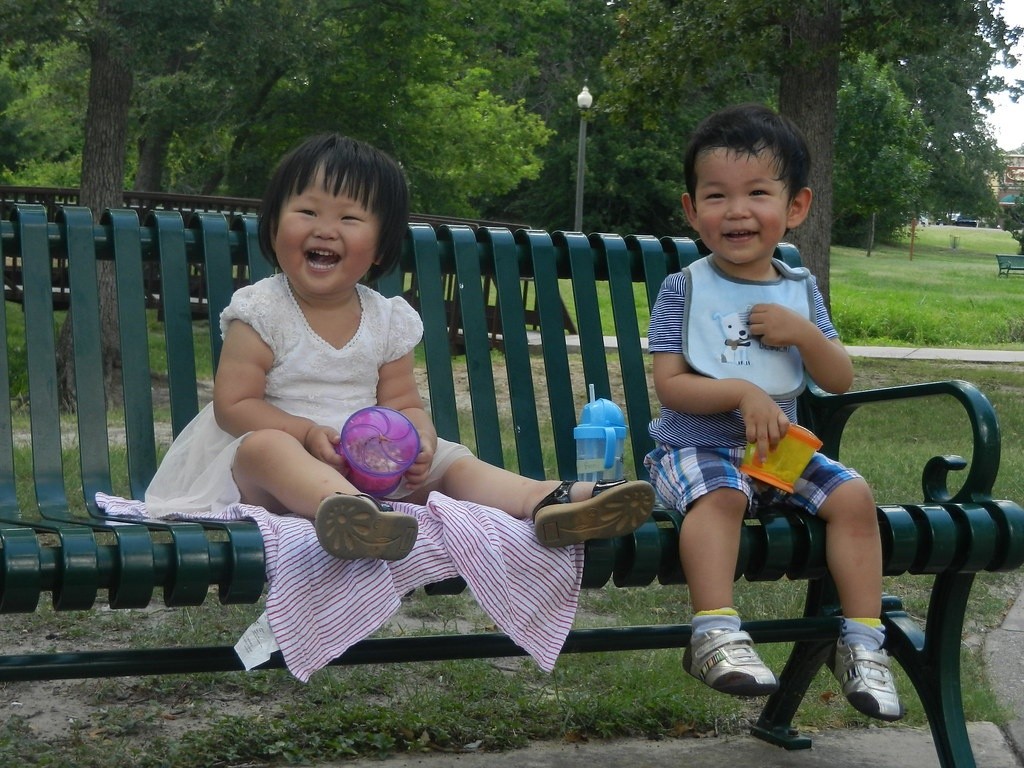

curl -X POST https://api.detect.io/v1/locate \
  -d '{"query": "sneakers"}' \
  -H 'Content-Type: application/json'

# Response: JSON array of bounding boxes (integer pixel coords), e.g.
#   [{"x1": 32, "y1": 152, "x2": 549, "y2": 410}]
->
[
  {"x1": 681, "y1": 607, "x2": 780, "y2": 698},
  {"x1": 828, "y1": 617, "x2": 904, "y2": 722}
]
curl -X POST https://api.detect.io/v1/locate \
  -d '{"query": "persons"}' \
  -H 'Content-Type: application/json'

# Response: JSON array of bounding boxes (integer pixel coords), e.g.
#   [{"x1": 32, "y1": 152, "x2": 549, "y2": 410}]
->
[
  {"x1": 145, "y1": 133, "x2": 658, "y2": 563},
  {"x1": 642, "y1": 100, "x2": 909, "y2": 724}
]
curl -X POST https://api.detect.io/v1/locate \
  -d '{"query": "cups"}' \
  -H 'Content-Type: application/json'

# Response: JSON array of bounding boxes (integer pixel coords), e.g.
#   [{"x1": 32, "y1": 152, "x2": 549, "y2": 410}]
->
[
  {"x1": 333, "y1": 405, "x2": 421, "y2": 499},
  {"x1": 741, "y1": 422, "x2": 824, "y2": 495}
]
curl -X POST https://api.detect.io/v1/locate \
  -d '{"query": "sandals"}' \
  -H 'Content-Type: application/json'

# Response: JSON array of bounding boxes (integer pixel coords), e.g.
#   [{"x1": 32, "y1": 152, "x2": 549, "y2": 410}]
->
[
  {"x1": 530, "y1": 479, "x2": 655, "y2": 547},
  {"x1": 314, "y1": 492, "x2": 419, "y2": 562}
]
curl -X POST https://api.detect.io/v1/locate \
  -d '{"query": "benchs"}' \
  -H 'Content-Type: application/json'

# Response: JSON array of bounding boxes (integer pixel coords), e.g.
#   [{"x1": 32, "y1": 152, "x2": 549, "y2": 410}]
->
[
  {"x1": 1, "y1": 196, "x2": 1024, "y2": 765},
  {"x1": 995, "y1": 254, "x2": 1024, "y2": 277}
]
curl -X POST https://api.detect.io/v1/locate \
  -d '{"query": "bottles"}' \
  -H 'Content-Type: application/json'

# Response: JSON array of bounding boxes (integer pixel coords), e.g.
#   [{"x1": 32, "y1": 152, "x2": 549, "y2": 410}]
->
[{"x1": 571, "y1": 382, "x2": 629, "y2": 482}]
